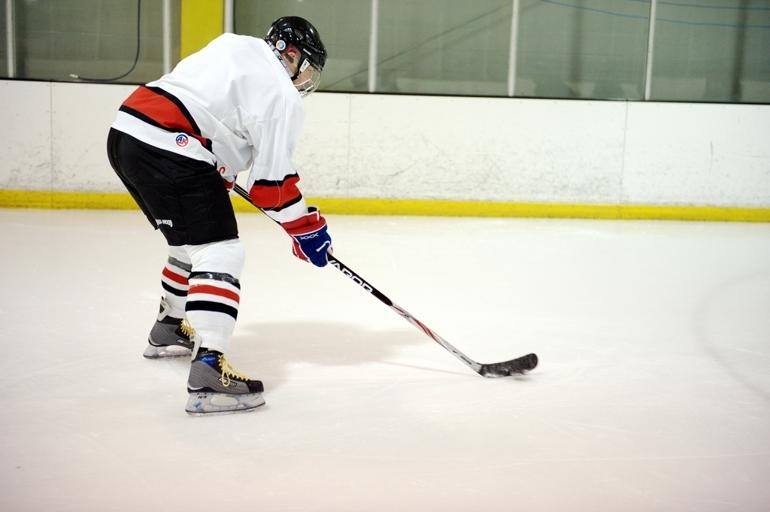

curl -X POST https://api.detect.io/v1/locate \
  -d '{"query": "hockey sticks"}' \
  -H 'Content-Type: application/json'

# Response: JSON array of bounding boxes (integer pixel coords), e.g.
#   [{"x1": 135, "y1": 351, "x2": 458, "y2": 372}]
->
[{"x1": 233, "y1": 182, "x2": 538, "y2": 377}]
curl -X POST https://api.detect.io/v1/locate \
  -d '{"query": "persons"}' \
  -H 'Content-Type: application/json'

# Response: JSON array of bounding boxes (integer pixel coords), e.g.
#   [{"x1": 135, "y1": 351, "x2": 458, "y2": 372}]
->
[{"x1": 105, "y1": 16, "x2": 336, "y2": 416}]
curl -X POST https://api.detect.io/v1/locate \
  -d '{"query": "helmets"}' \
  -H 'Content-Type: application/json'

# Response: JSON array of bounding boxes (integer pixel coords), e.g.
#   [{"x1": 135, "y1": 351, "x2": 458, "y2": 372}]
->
[{"x1": 264, "y1": 16, "x2": 326, "y2": 97}]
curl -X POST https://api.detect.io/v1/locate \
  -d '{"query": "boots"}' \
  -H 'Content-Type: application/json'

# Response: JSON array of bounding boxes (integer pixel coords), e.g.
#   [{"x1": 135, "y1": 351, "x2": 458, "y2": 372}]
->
[
  {"x1": 147, "y1": 297, "x2": 194, "y2": 349},
  {"x1": 187, "y1": 333, "x2": 265, "y2": 393}
]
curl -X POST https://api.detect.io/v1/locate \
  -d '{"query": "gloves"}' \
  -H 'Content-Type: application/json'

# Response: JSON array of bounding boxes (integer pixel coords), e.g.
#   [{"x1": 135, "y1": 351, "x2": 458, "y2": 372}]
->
[{"x1": 281, "y1": 206, "x2": 333, "y2": 268}]
